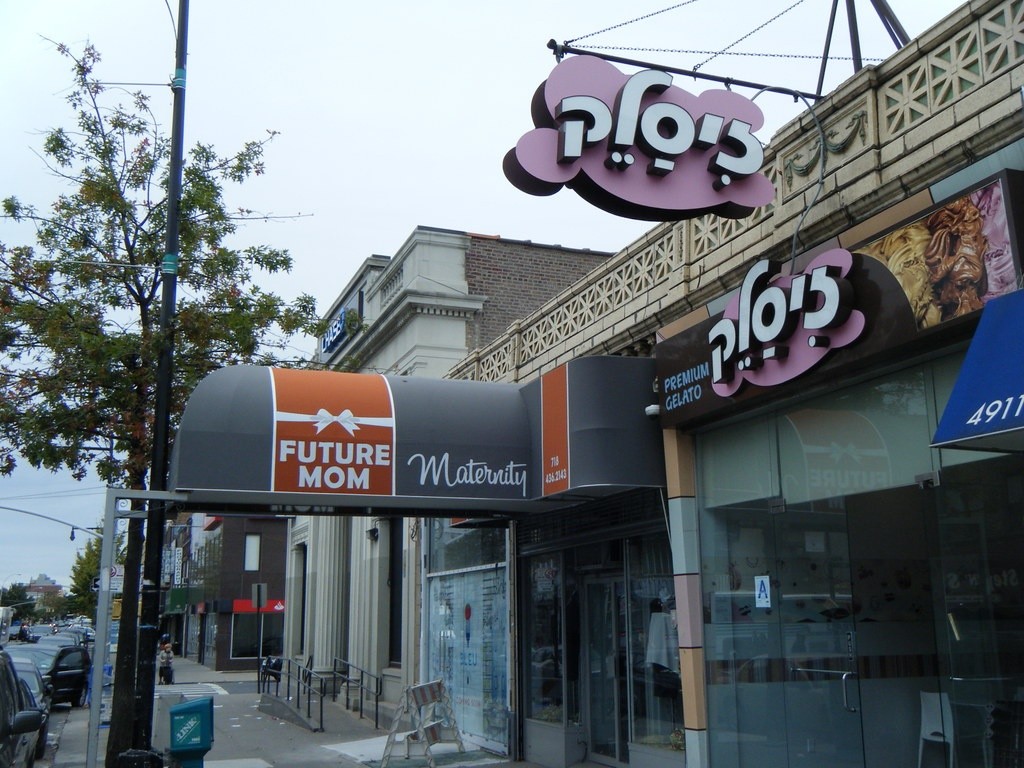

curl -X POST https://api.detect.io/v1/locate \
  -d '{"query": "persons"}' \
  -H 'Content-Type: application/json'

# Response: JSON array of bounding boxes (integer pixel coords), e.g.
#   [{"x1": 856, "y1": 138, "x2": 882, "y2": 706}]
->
[
  {"x1": 158, "y1": 643, "x2": 174, "y2": 684},
  {"x1": 18, "y1": 623, "x2": 27, "y2": 642}
]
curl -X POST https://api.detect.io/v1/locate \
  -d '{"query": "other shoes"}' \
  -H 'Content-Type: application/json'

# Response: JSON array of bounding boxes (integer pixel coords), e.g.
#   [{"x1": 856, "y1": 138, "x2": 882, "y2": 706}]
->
[{"x1": 158, "y1": 682, "x2": 163, "y2": 685}]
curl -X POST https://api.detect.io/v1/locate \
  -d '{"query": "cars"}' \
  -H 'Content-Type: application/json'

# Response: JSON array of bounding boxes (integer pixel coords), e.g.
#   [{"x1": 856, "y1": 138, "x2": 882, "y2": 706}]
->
[{"x1": 0, "y1": 616, "x2": 96, "y2": 768}]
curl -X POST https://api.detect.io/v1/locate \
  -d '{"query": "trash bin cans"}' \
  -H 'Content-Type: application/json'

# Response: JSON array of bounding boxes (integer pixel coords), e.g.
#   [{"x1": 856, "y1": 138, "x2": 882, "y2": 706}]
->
[{"x1": 87, "y1": 665, "x2": 113, "y2": 708}]
